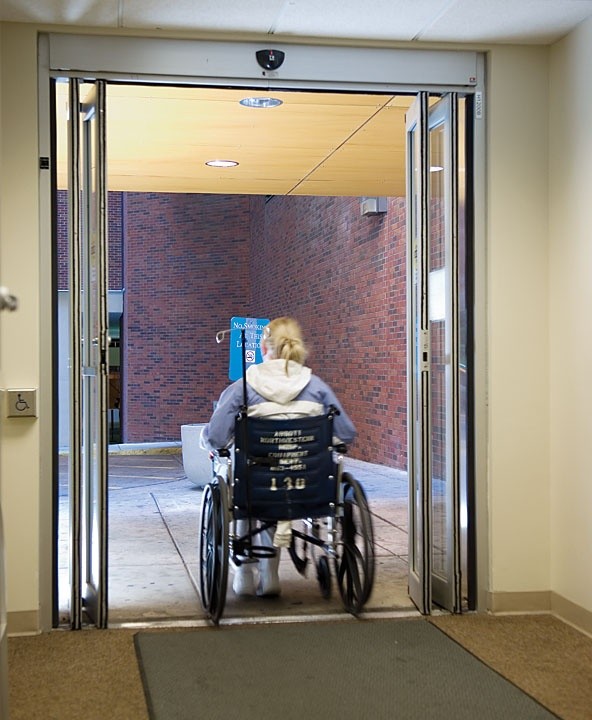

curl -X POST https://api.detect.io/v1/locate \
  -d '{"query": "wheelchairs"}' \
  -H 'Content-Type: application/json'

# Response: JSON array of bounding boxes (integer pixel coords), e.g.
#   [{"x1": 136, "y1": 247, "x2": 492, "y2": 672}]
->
[{"x1": 199, "y1": 403, "x2": 376, "y2": 626}]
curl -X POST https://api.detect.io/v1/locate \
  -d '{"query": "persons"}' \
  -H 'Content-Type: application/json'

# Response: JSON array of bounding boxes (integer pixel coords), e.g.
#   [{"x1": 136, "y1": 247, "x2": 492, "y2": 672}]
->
[{"x1": 199, "y1": 317, "x2": 358, "y2": 597}]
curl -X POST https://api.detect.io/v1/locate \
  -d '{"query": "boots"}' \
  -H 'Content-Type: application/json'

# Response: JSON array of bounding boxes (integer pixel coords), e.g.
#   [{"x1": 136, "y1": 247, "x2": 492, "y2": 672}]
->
[
  {"x1": 233, "y1": 555, "x2": 260, "y2": 595},
  {"x1": 254, "y1": 547, "x2": 281, "y2": 596}
]
